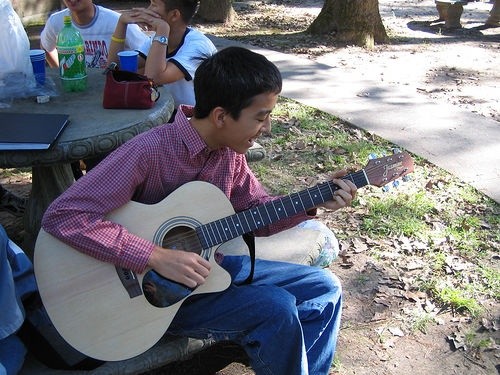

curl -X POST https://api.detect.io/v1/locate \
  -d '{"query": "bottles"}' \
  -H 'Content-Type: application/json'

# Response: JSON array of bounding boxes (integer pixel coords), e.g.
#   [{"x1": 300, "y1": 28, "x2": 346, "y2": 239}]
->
[{"x1": 56, "y1": 16, "x2": 87, "y2": 93}]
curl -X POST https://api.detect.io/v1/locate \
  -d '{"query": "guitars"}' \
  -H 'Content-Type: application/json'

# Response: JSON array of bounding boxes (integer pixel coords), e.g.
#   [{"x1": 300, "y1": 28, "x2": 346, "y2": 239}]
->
[{"x1": 33, "y1": 147, "x2": 415, "y2": 362}]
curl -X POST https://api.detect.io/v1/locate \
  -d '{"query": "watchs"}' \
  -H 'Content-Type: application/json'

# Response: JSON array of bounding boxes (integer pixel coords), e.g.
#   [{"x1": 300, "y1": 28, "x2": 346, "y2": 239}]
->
[{"x1": 154, "y1": 36, "x2": 168, "y2": 44}]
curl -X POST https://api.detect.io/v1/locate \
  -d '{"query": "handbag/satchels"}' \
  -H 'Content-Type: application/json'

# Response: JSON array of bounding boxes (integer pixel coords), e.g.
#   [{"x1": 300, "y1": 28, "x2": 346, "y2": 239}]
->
[
  {"x1": 103, "y1": 69, "x2": 160, "y2": 109},
  {"x1": 0, "y1": 0, "x2": 36, "y2": 96}
]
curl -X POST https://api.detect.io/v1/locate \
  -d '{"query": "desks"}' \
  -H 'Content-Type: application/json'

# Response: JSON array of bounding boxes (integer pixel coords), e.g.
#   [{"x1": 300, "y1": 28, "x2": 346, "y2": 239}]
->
[{"x1": 0, "y1": 66, "x2": 176, "y2": 239}]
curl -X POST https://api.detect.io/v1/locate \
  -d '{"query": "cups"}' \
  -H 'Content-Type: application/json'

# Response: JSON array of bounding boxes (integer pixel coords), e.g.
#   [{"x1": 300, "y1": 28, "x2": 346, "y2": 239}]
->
[
  {"x1": 30, "y1": 49, "x2": 46, "y2": 84},
  {"x1": 117, "y1": 51, "x2": 139, "y2": 73}
]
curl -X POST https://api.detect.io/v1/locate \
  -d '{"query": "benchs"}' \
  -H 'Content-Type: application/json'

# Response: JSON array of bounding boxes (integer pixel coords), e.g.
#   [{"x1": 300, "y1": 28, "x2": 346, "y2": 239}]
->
[{"x1": 15, "y1": 220, "x2": 340, "y2": 375}]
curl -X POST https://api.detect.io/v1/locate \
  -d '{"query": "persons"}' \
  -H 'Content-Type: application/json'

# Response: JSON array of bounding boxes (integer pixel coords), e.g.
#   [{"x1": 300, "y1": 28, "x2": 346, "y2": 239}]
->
[
  {"x1": 107, "y1": 0, "x2": 218, "y2": 124},
  {"x1": 40, "y1": 0, "x2": 151, "y2": 70},
  {"x1": 41, "y1": 47, "x2": 357, "y2": 375}
]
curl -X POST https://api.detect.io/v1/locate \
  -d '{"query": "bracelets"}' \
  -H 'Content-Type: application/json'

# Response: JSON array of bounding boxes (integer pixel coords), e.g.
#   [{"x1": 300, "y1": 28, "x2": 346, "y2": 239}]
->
[{"x1": 112, "y1": 37, "x2": 125, "y2": 42}]
